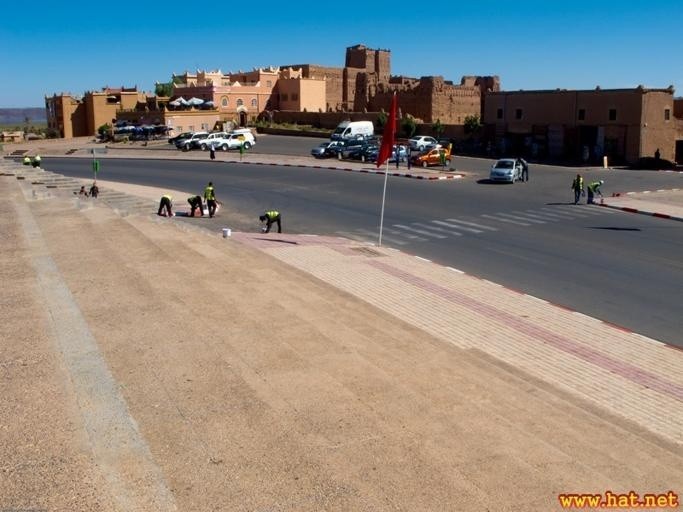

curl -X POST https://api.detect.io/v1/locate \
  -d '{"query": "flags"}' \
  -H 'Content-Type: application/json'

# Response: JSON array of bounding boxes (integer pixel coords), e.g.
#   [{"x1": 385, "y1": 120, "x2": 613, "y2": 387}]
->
[{"x1": 376, "y1": 94, "x2": 396, "y2": 170}]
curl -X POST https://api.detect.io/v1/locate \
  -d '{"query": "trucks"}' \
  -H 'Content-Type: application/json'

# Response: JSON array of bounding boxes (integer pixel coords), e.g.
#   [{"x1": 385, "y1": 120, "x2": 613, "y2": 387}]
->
[{"x1": 331, "y1": 120, "x2": 375, "y2": 142}]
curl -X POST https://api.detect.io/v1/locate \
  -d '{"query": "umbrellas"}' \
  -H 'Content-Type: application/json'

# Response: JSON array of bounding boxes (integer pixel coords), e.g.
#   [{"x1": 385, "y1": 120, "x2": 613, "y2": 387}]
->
[
  {"x1": 168, "y1": 95, "x2": 214, "y2": 108},
  {"x1": 97, "y1": 117, "x2": 173, "y2": 141}
]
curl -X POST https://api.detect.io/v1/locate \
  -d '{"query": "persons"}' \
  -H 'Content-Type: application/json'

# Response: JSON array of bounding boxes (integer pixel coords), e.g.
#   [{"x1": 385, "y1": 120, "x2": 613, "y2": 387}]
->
[
  {"x1": 571, "y1": 172, "x2": 585, "y2": 205},
  {"x1": 156, "y1": 193, "x2": 177, "y2": 217},
  {"x1": 23, "y1": 155, "x2": 31, "y2": 166},
  {"x1": 438, "y1": 148, "x2": 446, "y2": 170},
  {"x1": 209, "y1": 142, "x2": 215, "y2": 160},
  {"x1": 259, "y1": 209, "x2": 281, "y2": 232},
  {"x1": 187, "y1": 195, "x2": 204, "y2": 218},
  {"x1": 88, "y1": 181, "x2": 99, "y2": 198},
  {"x1": 587, "y1": 180, "x2": 604, "y2": 204},
  {"x1": 203, "y1": 181, "x2": 221, "y2": 219},
  {"x1": 78, "y1": 186, "x2": 88, "y2": 200},
  {"x1": 517, "y1": 157, "x2": 529, "y2": 183},
  {"x1": 31, "y1": 154, "x2": 41, "y2": 169}
]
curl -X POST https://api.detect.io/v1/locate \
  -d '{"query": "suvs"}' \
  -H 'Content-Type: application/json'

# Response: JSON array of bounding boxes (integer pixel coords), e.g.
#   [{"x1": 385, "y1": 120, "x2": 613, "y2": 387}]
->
[{"x1": 167, "y1": 127, "x2": 258, "y2": 151}]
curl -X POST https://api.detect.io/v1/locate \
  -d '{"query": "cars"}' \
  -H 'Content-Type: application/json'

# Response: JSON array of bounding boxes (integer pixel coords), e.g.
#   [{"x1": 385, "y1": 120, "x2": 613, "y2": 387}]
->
[
  {"x1": 311, "y1": 131, "x2": 453, "y2": 168},
  {"x1": 488, "y1": 158, "x2": 524, "y2": 184}
]
